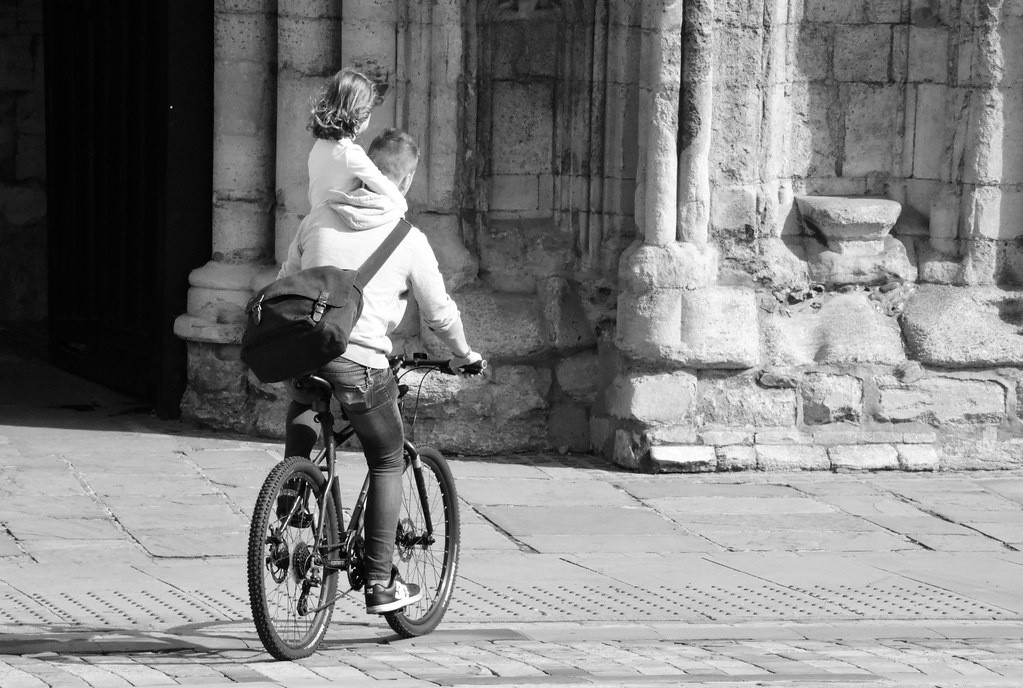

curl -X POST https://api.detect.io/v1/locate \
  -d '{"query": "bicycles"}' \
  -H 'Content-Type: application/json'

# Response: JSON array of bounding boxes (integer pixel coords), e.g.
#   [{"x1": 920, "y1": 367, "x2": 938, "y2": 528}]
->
[{"x1": 247, "y1": 353, "x2": 490, "y2": 660}]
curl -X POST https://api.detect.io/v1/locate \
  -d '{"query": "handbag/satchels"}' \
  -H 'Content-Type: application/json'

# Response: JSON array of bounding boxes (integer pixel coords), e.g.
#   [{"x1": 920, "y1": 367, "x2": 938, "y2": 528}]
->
[{"x1": 240, "y1": 266, "x2": 364, "y2": 383}]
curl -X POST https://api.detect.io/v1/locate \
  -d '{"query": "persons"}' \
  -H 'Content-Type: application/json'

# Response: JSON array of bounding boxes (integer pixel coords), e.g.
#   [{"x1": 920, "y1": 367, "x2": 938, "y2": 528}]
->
[
  {"x1": 275, "y1": 128, "x2": 482, "y2": 616},
  {"x1": 307, "y1": 67, "x2": 409, "y2": 218}
]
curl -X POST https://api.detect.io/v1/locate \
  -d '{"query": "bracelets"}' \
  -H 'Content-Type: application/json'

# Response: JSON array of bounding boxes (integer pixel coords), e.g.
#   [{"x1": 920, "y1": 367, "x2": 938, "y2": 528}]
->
[{"x1": 451, "y1": 344, "x2": 472, "y2": 359}]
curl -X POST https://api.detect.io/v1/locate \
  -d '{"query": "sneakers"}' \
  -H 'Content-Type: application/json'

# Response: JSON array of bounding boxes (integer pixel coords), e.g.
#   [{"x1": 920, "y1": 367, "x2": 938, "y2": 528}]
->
[
  {"x1": 276, "y1": 479, "x2": 308, "y2": 524},
  {"x1": 364, "y1": 563, "x2": 423, "y2": 613}
]
